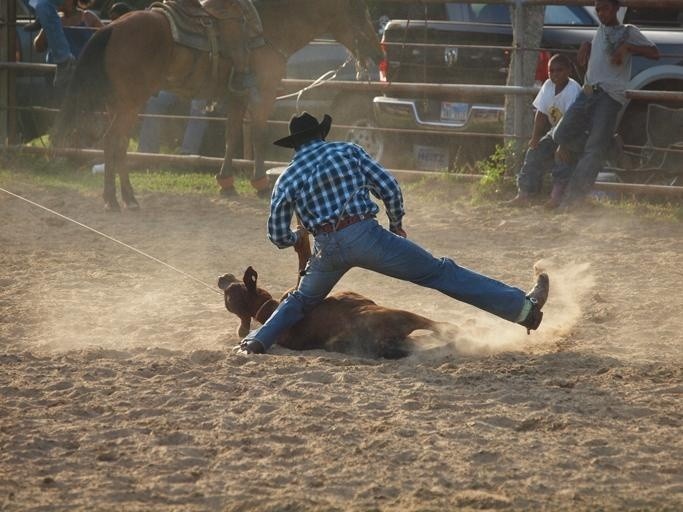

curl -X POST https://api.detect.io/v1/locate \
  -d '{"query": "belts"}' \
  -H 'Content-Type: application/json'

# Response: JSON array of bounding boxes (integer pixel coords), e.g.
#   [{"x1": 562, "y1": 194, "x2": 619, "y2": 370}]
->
[{"x1": 312, "y1": 210, "x2": 379, "y2": 239}]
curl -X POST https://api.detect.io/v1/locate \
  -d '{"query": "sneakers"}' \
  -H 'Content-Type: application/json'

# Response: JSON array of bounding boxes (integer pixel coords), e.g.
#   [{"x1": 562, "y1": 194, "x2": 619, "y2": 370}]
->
[
  {"x1": 541, "y1": 184, "x2": 563, "y2": 209},
  {"x1": 52, "y1": 51, "x2": 78, "y2": 87},
  {"x1": 495, "y1": 193, "x2": 530, "y2": 210}
]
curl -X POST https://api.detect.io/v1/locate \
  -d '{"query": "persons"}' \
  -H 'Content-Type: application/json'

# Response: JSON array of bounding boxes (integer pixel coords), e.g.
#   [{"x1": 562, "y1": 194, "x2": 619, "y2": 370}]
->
[
  {"x1": 231, "y1": 105, "x2": 552, "y2": 354},
  {"x1": 543, "y1": 2, "x2": 658, "y2": 209},
  {"x1": 498, "y1": 51, "x2": 582, "y2": 209},
  {"x1": 9, "y1": 0, "x2": 271, "y2": 161}
]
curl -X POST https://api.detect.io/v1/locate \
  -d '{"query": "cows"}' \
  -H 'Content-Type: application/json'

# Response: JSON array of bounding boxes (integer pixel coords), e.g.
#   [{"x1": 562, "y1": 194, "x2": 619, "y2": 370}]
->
[{"x1": 217, "y1": 266, "x2": 460, "y2": 361}]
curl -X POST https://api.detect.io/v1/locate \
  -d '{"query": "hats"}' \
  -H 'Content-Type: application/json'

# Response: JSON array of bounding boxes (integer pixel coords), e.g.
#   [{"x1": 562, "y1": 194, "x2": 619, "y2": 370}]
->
[{"x1": 272, "y1": 111, "x2": 332, "y2": 149}]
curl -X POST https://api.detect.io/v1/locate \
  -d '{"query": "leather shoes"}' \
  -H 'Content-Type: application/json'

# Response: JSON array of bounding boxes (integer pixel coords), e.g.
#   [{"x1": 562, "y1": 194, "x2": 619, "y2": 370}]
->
[
  {"x1": 231, "y1": 339, "x2": 265, "y2": 355},
  {"x1": 518, "y1": 271, "x2": 550, "y2": 334}
]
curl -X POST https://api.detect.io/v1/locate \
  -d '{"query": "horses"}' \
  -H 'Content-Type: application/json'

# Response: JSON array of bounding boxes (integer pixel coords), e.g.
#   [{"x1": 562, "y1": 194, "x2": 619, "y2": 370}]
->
[{"x1": 40, "y1": 1, "x2": 388, "y2": 215}]
